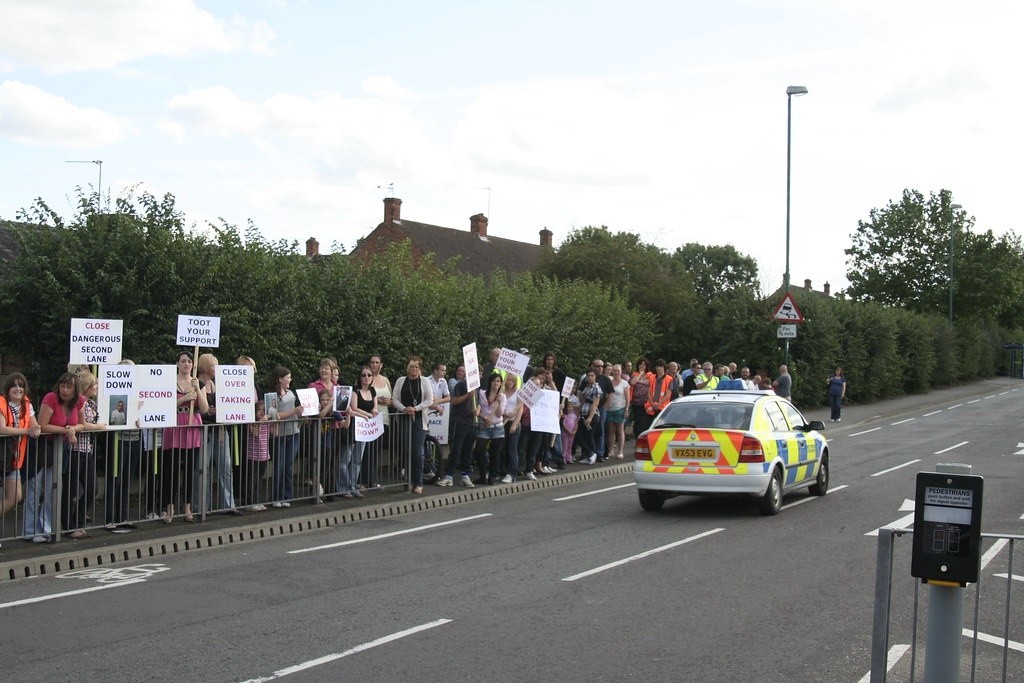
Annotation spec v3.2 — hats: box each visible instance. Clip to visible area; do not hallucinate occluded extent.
[518,347,530,356]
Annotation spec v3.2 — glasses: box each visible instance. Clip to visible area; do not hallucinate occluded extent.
[361,373,372,377]
[704,367,710,370]
[595,365,604,368]
[695,367,701,369]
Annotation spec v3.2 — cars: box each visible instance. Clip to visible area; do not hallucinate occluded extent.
[632,392,832,516]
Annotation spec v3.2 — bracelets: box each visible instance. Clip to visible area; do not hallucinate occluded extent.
[75,427,77,432]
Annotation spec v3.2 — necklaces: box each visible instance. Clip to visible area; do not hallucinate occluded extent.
[409,377,419,405]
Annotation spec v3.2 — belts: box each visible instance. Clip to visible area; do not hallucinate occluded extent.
[480,422,504,428]
[177,406,200,414]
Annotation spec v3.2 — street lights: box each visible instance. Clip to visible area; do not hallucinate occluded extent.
[783,83,810,368]
[949,202,963,324]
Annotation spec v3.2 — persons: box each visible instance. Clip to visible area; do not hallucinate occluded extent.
[667,359,775,402]
[426,348,674,488]
[392,356,434,492]
[773,365,791,402]
[827,367,846,421]
[0,351,280,543]
[306,355,392,501]
[336,388,349,411]
[111,401,126,425]
[268,367,321,507]
[269,399,277,419]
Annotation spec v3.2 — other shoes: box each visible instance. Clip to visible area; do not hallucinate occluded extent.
[31,447,625,544]
[830,419,835,422]
[836,418,841,422]
